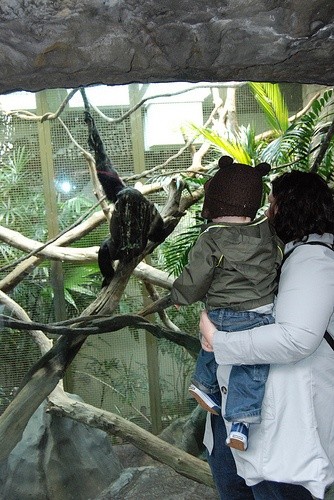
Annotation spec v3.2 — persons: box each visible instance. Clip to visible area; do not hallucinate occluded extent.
[184,167,333,500]
[172,158,282,451]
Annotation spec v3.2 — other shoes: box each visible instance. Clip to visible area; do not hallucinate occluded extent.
[225,420,250,452]
[187,383,222,417]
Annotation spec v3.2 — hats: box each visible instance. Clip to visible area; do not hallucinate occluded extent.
[201,155,271,217]
